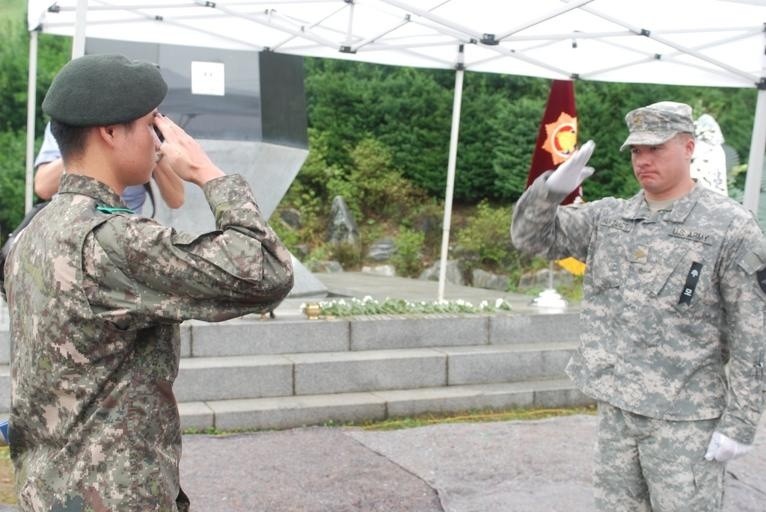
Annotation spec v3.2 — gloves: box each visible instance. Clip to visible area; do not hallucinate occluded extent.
[704,431,752,463]
[546,140,595,195]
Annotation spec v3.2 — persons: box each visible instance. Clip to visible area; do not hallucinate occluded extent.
[0,54,296,511]
[508,102,766,511]
[33,107,186,217]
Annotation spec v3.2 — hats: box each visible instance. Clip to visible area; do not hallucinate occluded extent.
[620,100,694,153]
[42,54,168,126]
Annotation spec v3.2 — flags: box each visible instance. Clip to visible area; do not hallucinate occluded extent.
[526,74,581,207]
